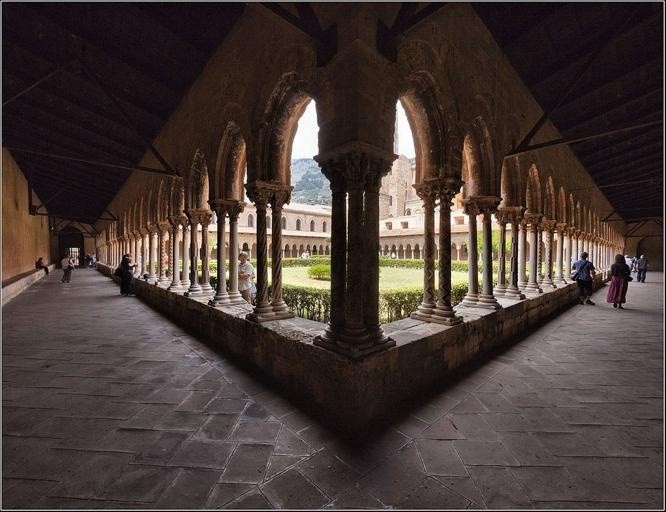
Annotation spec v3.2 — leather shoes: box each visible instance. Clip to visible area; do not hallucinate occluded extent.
[614,304,617,308]
[620,306,624,309]
[587,301,595,305]
[579,301,584,305]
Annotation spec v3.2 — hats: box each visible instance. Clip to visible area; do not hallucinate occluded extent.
[640,254,646,259]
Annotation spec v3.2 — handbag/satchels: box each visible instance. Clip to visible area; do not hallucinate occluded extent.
[570,272,580,281]
[624,274,633,283]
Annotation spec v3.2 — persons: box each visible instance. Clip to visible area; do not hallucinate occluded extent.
[637,254,650,282]
[237,251,256,304]
[60,254,76,283]
[571,251,596,306]
[36,256,48,275]
[625,255,639,273]
[78,252,97,268]
[300,250,310,259]
[116,253,138,298]
[607,254,633,309]
